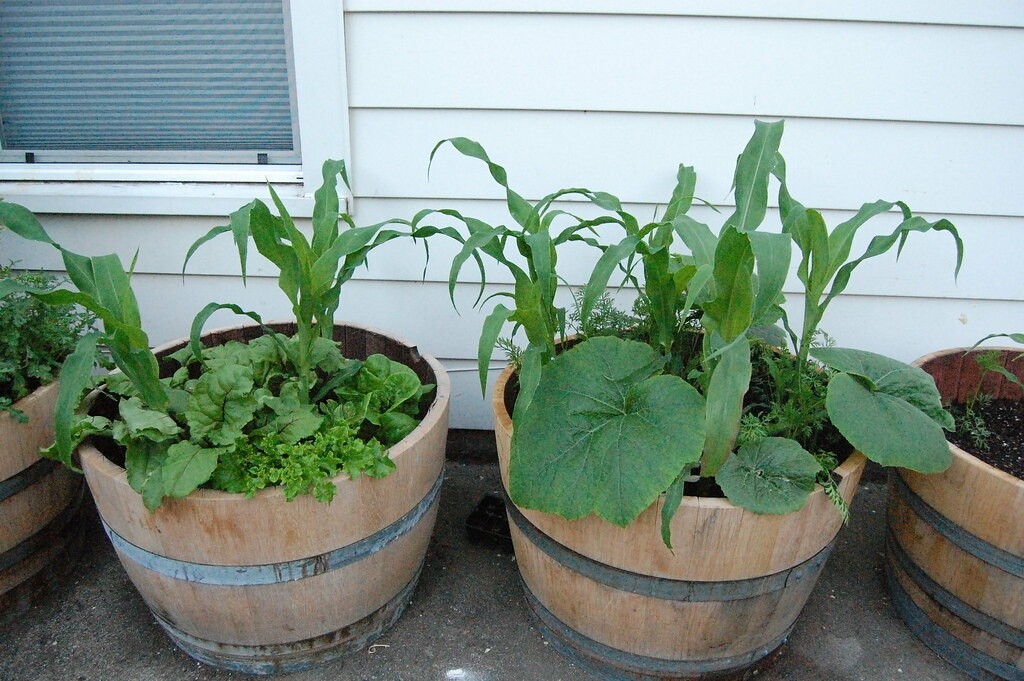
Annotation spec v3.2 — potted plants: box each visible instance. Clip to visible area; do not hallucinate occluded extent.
[0,199,117,612]
[408,118,964,680]
[879,333,1024,680]
[1,158,451,674]
